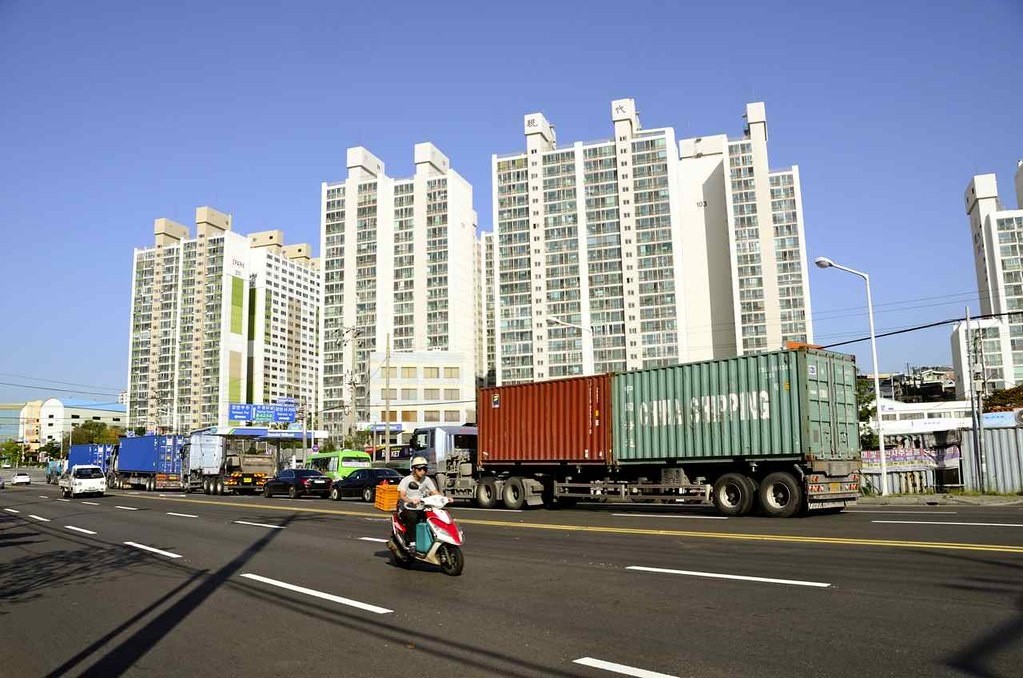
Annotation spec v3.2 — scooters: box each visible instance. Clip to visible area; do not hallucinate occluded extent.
[385,481,464,577]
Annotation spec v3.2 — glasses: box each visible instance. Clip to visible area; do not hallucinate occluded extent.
[416,466,428,471]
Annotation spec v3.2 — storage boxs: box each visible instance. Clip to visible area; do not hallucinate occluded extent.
[375,485,400,512]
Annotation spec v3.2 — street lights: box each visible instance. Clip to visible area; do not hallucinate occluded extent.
[815,257,888,498]
[545,318,594,374]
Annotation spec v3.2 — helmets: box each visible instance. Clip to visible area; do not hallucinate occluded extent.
[411,457,428,468]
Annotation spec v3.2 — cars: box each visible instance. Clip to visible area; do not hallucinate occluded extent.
[263,469,332,499]
[11,472,31,485]
[0,476,5,489]
[329,468,405,502]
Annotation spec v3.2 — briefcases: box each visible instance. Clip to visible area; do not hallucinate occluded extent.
[417,523,433,553]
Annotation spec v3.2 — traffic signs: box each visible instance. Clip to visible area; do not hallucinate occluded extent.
[273,405,295,422]
[252,403,275,423]
[228,403,252,421]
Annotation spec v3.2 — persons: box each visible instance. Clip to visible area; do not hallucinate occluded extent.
[397,457,454,549]
[248,444,257,454]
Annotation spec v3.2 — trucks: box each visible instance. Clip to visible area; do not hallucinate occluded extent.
[43,442,115,485]
[58,464,106,499]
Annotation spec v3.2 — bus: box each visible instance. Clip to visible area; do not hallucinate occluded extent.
[304,449,372,483]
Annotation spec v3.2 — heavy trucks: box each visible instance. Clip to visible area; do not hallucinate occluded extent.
[105,434,185,491]
[409,345,862,518]
[179,434,276,495]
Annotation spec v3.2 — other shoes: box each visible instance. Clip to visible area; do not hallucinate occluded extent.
[409,545,416,552]
